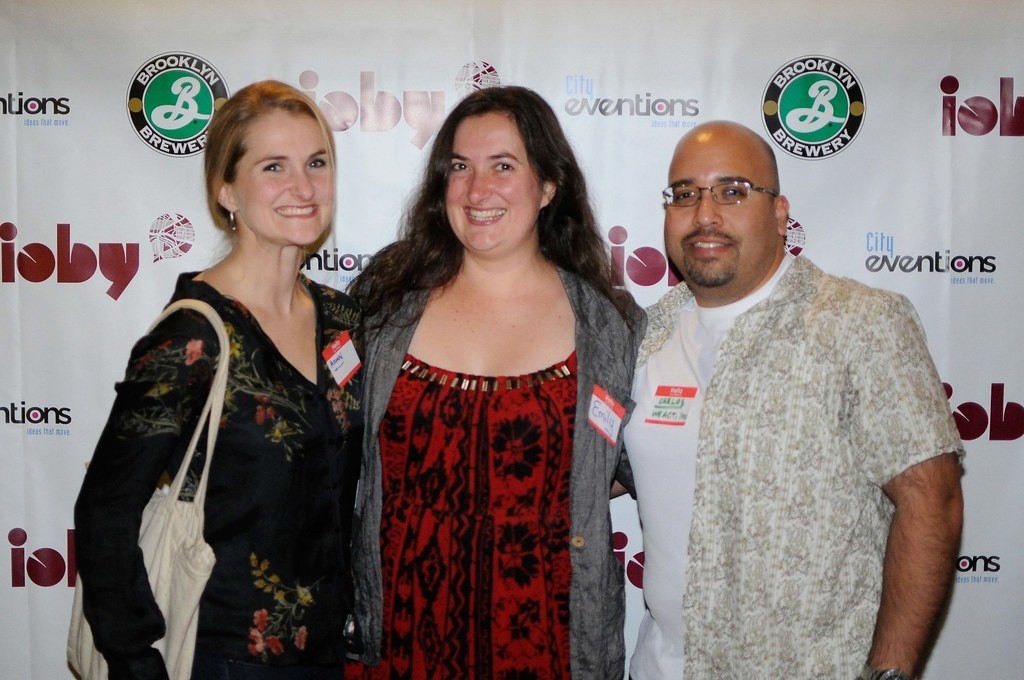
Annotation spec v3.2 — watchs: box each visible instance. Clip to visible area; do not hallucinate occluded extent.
[859,662,910,680]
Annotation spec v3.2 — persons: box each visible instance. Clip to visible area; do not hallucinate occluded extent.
[74,82,364,680]
[610,121,964,680]
[346,85,646,680]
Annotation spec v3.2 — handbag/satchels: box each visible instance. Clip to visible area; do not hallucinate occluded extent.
[66,299,230,680]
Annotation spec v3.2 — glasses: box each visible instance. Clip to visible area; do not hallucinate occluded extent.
[662,181,779,207]
